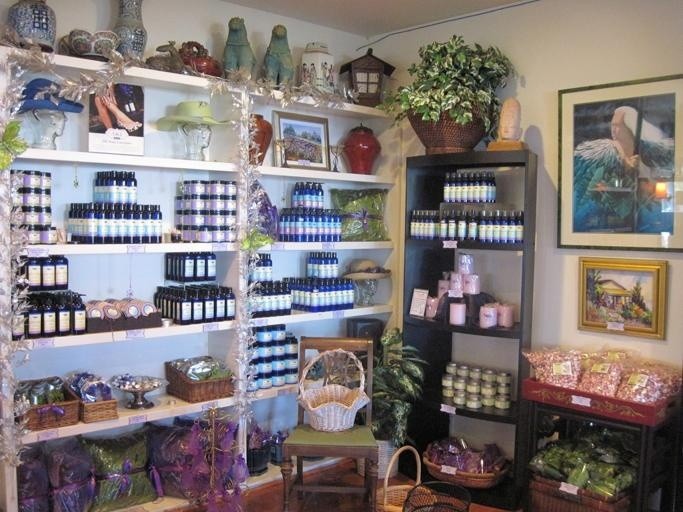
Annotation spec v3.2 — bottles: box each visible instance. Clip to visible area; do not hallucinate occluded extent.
[27,295,41,338]
[409,171,524,244]
[266,430,288,465]
[442,361,512,410]
[41,259,55,289]
[56,293,70,334]
[171,178,237,242]
[42,294,56,337]
[12,296,25,341]
[248,324,298,388]
[249,251,353,317]
[26,256,41,291]
[73,293,87,332]
[279,181,342,242]
[53,255,68,290]
[154,253,235,324]
[7,169,56,244]
[13,256,26,291]
[67,170,162,243]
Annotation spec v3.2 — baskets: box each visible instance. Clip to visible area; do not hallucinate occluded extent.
[531,474,637,512]
[165,356,235,404]
[402,481,473,512]
[17,374,82,429]
[297,348,370,432]
[406,102,492,153]
[376,443,434,512]
[424,448,513,489]
[80,401,120,423]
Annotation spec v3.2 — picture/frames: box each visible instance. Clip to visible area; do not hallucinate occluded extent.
[556,72,683,253]
[577,254,669,342]
[270,109,333,172]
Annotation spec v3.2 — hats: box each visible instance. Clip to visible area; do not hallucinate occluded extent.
[342,258,391,281]
[156,99,232,132]
[19,77,82,114]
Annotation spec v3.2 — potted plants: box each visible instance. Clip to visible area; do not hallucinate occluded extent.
[375,35,517,156]
[356,328,431,480]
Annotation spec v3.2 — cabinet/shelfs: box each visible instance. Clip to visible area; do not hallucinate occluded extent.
[400,148,538,508]
[0,46,407,508]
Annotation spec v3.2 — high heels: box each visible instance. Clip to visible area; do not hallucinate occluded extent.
[115,119,142,132]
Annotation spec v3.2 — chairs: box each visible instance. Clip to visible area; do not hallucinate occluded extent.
[279,336,381,507]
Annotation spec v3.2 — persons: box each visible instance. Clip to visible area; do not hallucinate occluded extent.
[94,85,142,131]
